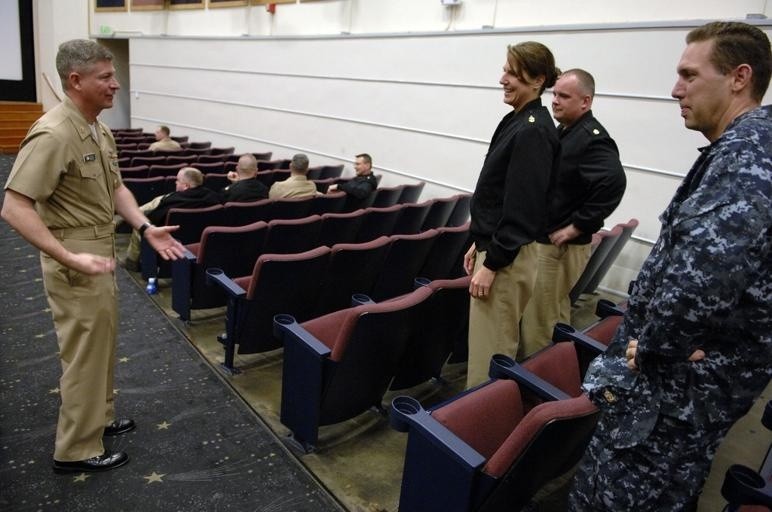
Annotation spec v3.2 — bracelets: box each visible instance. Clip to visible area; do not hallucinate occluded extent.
[138,222,154,235]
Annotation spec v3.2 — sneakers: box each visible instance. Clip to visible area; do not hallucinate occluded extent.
[120,256,138,272]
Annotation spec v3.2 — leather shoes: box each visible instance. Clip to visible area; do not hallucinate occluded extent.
[53,448,129,475]
[102,418,137,436]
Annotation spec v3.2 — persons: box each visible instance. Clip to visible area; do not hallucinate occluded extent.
[1,36,188,474]
[325,153,377,201]
[124,167,217,269]
[519,67,628,352]
[267,153,317,200]
[217,153,267,204]
[148,123,181,151]
[564,22,770,510]
[465,39,559,397]
